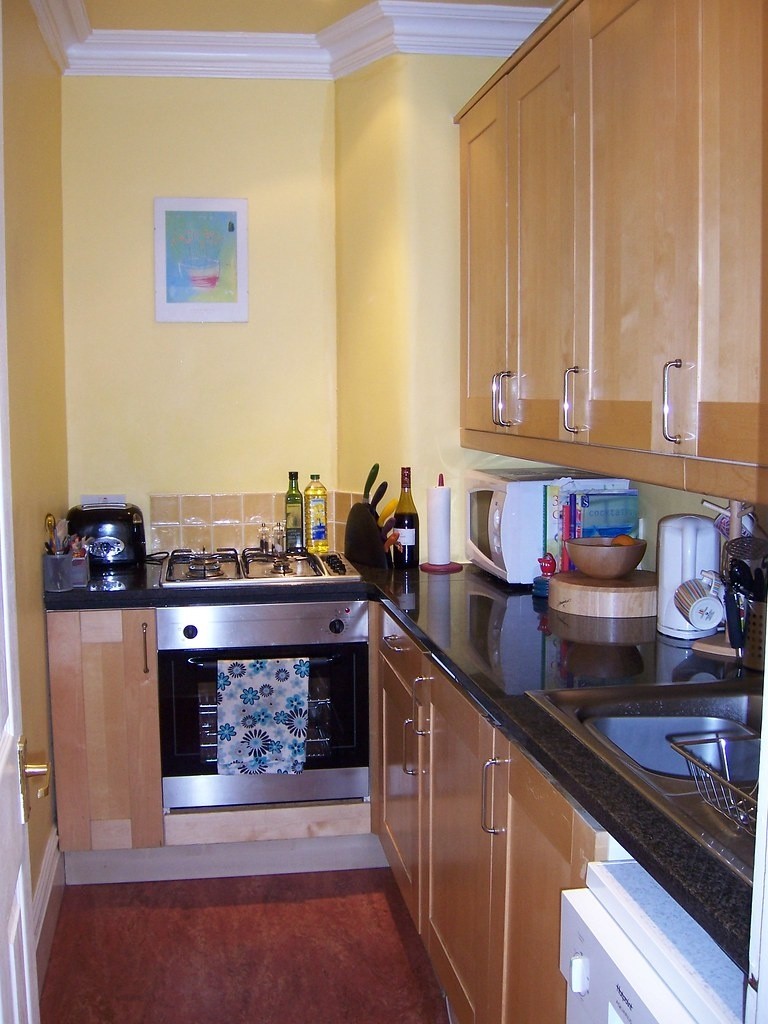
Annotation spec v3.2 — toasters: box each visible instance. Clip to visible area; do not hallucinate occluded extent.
[68,503,147,569]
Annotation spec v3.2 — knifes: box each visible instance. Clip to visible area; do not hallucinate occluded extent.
[362,463,399,552]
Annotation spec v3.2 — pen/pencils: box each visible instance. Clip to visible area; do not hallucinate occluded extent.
[44,536,73,590]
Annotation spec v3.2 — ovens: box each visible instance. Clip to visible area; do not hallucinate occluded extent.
[156,599,370,813]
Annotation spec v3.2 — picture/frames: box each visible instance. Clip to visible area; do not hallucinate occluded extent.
[154,197,248,324]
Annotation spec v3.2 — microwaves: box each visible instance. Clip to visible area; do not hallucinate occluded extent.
[464,567,567,696]
[463,468,630,584]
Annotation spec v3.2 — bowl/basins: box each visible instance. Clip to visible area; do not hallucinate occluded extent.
[563,643,644,677]
[565,538,647,579]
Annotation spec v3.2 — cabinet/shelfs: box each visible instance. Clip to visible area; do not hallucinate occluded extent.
[454,0,768,506]
[372,607,633,1024]
[45,607,165,852]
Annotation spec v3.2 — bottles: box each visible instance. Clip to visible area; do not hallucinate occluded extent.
[394,569,420,623]
[259,523,269,551]
[272,522,284,551]
[393,467,419,568]
[285,471,304,550]
[304,475,329,553]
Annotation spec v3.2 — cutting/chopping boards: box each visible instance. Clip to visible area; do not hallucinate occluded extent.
[547,572,657,618]
[548,609,657,645]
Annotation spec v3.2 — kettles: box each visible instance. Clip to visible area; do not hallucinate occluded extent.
[655,633,724,684]
[656,514,720,638]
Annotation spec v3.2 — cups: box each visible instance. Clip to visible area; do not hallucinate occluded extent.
[673,570,724,630]
[713,505,768,541]
[44,553,73,593]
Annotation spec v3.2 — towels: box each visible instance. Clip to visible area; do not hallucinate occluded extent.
[216,657,310,776]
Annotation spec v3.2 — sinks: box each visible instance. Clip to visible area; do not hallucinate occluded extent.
[522,677,765,798]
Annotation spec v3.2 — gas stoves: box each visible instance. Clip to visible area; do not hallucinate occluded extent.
[159,544,365,605]
